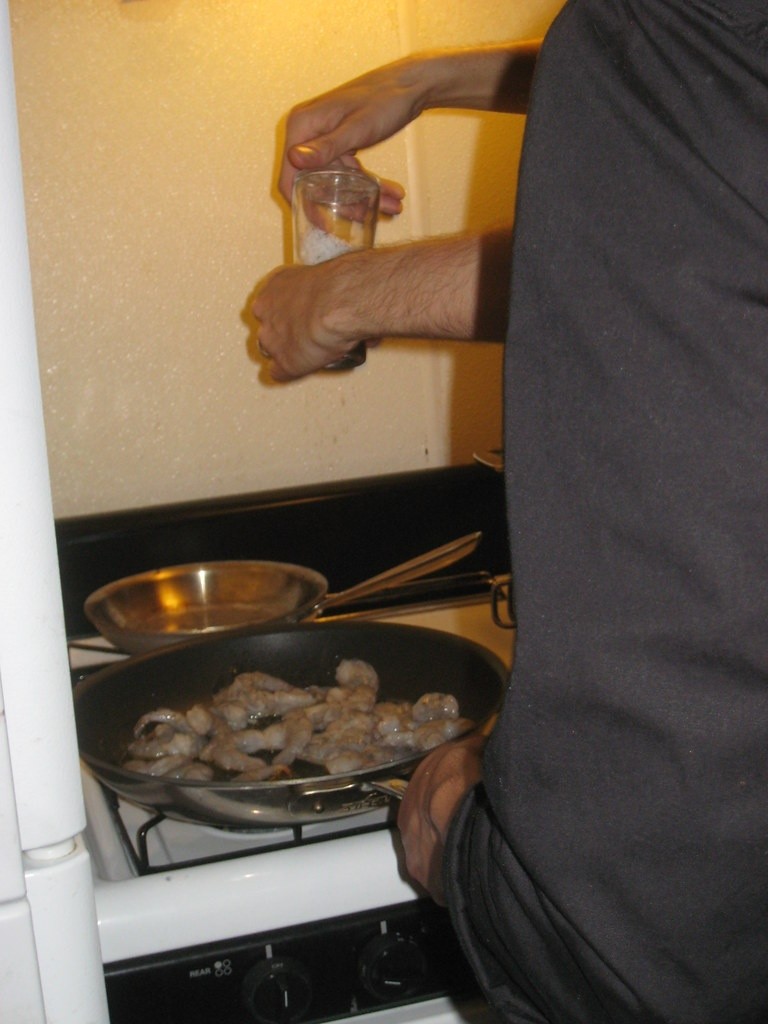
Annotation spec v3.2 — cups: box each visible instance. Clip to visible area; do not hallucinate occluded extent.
[292,167,379,370]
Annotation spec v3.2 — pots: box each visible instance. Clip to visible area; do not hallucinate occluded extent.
[84,531,482,656]
[73,620,510,827]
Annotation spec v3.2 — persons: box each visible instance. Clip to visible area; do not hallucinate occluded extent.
[397,0,768,1024]
[253,38,544,383]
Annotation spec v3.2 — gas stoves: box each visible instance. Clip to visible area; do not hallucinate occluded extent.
[53,463,507,1024]
